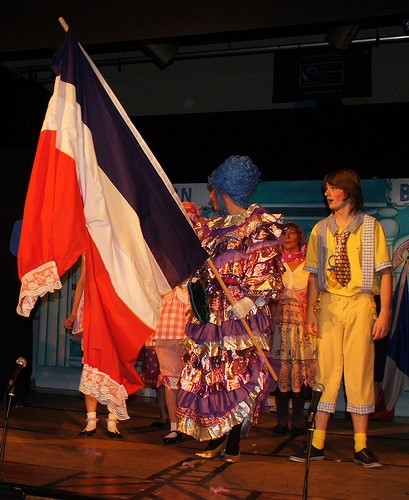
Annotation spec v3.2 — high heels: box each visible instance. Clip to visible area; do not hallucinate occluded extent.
[224,443,240,463]
[196,438,227,459]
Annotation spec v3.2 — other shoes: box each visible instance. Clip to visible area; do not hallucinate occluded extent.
[162,431,182,444]
[150,421,169,429]
[289,443,325,462]
[104,428,123,440]
[291,425,305,435]
[79,428,96,437]
[353,448,383,468]
[274,424,288,435]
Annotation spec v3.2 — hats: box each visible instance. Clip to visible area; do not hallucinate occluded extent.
[206,154,262,216]
[282,222,305,244]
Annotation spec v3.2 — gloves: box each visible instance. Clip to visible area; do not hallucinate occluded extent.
[227,296,254,320]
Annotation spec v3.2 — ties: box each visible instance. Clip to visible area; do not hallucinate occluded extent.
[333,231,351,287]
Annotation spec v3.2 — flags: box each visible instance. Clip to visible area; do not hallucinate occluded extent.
[18,30,211,422]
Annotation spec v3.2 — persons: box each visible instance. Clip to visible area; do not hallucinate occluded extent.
[65,154,393,468]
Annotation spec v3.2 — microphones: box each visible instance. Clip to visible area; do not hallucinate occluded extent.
[8,357,26,386]
[308,383,325,423]
